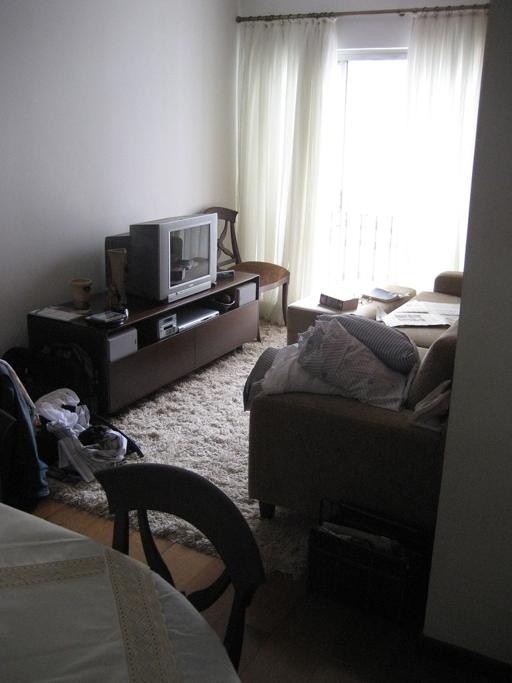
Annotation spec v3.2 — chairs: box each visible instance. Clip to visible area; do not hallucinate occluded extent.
[106,464,264,673]
[204,207,291,341]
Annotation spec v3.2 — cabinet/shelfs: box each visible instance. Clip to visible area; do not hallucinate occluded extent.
[29,270,258,414]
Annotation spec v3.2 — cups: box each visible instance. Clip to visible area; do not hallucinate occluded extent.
[70,277,93,312]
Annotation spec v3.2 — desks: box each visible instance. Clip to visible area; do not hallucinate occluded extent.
[1,500,243,681]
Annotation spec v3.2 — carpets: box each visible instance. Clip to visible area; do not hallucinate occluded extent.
[42,317,308,581]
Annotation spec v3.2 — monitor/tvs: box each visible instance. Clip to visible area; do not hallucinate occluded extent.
[104,212,219,304]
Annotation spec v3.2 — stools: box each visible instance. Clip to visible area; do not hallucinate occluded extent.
[287,286,415,346]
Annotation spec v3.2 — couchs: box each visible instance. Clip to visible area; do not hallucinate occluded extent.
[250,271,465,552]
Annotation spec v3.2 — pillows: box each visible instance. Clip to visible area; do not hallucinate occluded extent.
[318,313,421,376]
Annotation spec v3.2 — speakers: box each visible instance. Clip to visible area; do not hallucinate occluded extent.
[233,281,257,307]
[107,327,138,363]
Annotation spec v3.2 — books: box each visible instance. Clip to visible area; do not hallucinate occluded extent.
[318,289,361,312]
[364,285,410,302]
[170,266,186,280]
[174,258,196,269]
